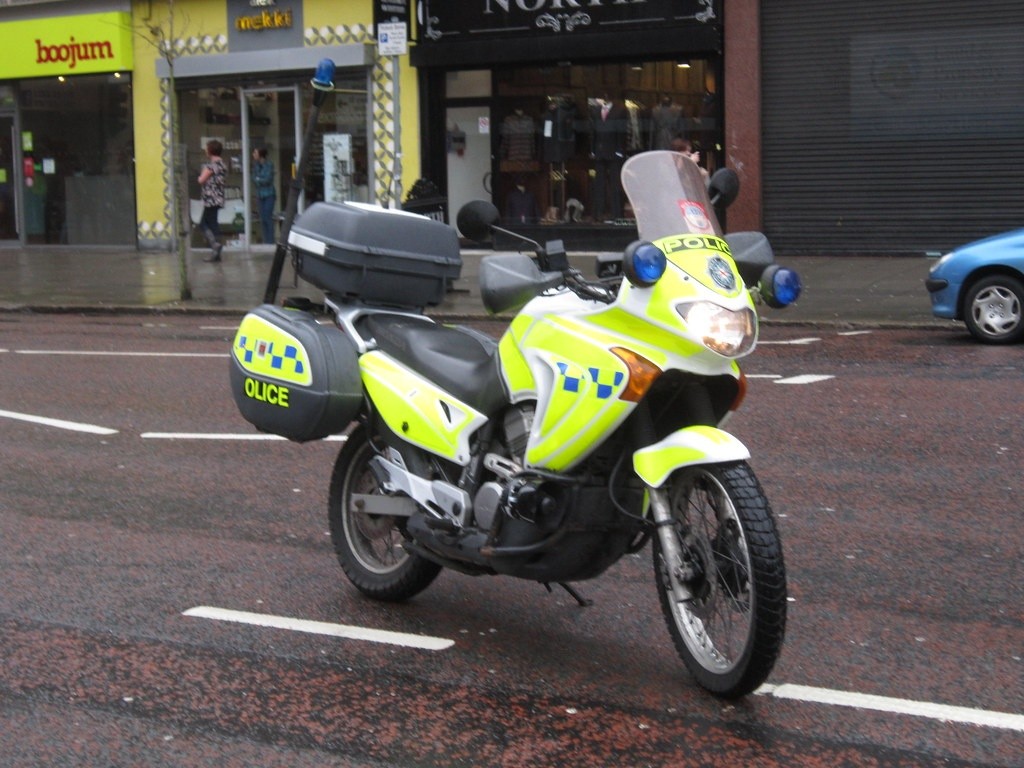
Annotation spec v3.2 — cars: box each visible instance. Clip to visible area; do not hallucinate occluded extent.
[925,226,1024,344]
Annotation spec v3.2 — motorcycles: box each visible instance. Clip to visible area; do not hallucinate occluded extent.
[229,149,801,699]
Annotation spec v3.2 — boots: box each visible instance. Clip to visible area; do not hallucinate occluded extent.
[203,229,222,261]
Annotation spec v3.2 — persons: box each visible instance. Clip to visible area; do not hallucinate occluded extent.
[251,147,275,244]
[501,105,538,223]
[197,140,228,262]
[651,97,684,151]
[588,89,628,222]
